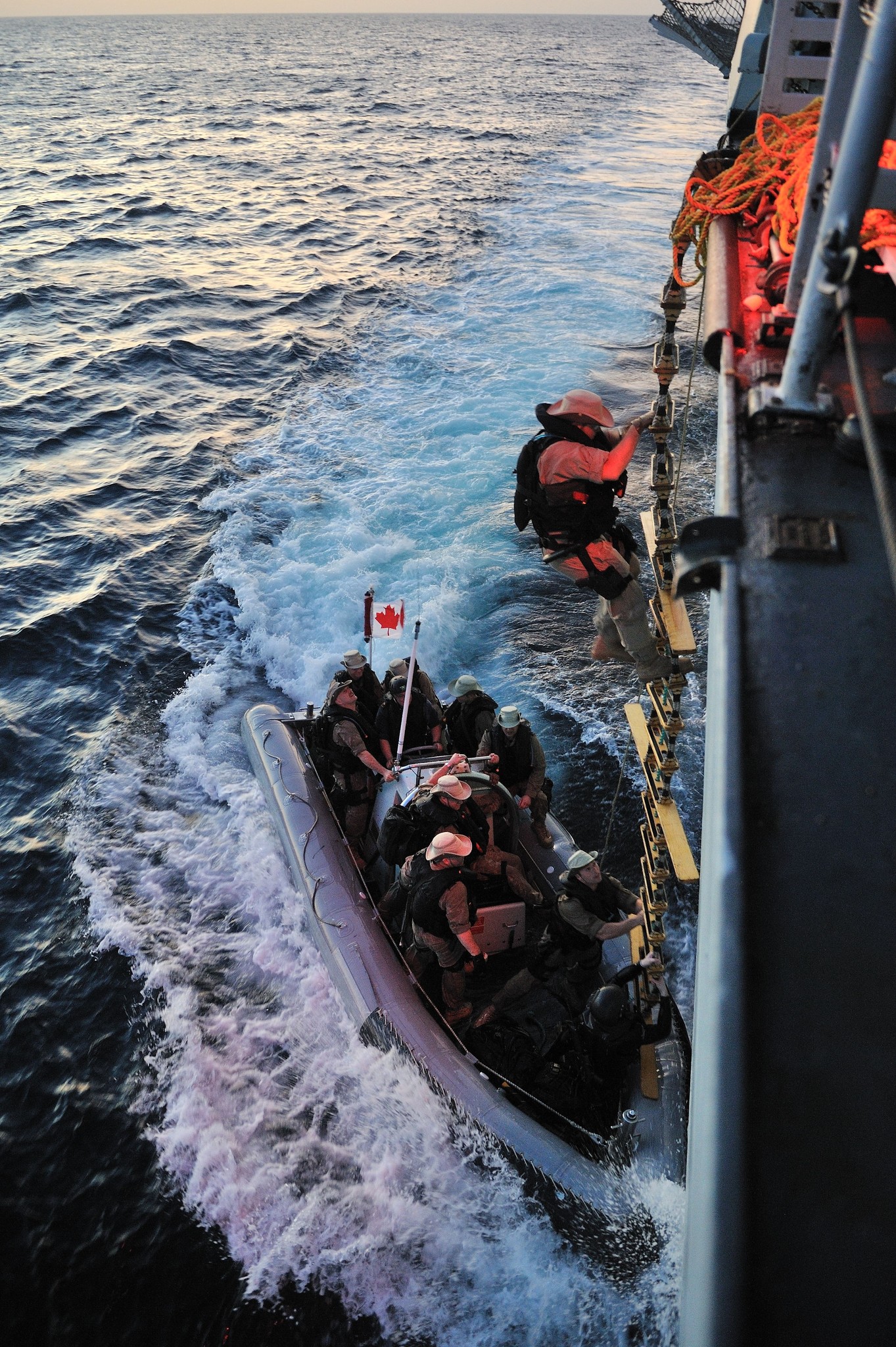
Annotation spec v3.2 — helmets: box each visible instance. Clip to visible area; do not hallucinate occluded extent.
[590,984,628,1027]
[389,675,408,694]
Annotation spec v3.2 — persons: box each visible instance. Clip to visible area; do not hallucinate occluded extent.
[441,673,500,763]
[373,752,477,927]
[373,675,443,770]
[378,657,442,725]
[477,705,556,849]
[407,829,490,1024]
[447,789,545,911]
[330,650,385,729]
[327,680,397,871]
[528,848,649,1011]
[556,949,675,1126]
[532,389,694,683]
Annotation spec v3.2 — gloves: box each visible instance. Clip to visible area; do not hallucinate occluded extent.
[630,411,656,434]
[471,951,486,976]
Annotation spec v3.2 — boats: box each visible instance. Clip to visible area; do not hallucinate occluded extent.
[238,585,690,1237]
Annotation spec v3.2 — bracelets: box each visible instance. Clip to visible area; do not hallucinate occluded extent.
[445,762,451,769]
[432,741,441,744]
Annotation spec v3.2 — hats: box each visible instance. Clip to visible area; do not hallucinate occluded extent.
[327,680,352,706]
[426,832,472,860]
[430,775,472,800]
[546,389,614,428]
[385,658,408,678]
[340,650,367,669]
[448,675,484,698]
[567,850,598,877]
[497,706,521,728]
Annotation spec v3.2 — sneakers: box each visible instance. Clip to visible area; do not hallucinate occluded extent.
[473,1004,499,1029]
[637,656,694,684]
[346,845,367,870]
[592,636,636,662]
[530,819,553,848]
[444,1001,473,1025]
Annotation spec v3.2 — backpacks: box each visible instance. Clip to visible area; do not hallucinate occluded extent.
[376,803,439,867]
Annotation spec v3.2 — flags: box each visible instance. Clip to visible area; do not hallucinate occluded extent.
[365,598,406,642]
